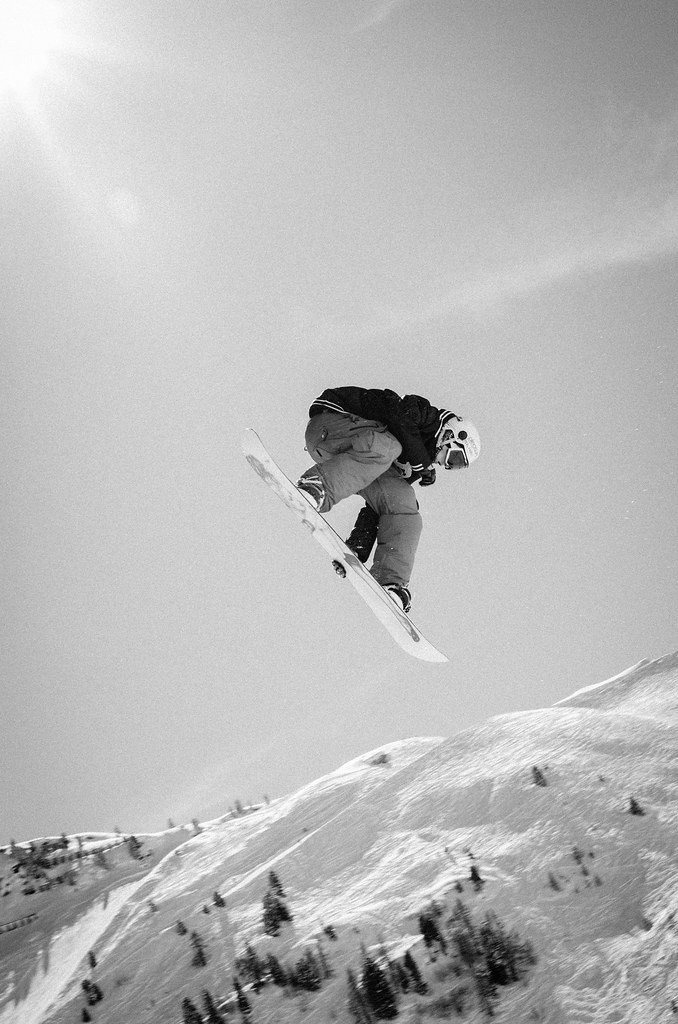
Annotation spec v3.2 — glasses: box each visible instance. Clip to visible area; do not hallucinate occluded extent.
[445,447,468,471]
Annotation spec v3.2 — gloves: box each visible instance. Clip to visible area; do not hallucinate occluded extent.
[332,560,346,578]
[419,464,436,487]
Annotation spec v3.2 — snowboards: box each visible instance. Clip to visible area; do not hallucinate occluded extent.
[235,427,454,667]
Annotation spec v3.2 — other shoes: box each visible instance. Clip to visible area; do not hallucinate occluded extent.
[383,583,413,612]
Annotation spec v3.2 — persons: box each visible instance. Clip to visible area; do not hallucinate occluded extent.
[295,385,481,613]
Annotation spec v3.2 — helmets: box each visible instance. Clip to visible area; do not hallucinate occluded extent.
[436,416,481,468]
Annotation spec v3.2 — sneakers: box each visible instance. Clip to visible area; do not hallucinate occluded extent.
[297,481,325,509]
